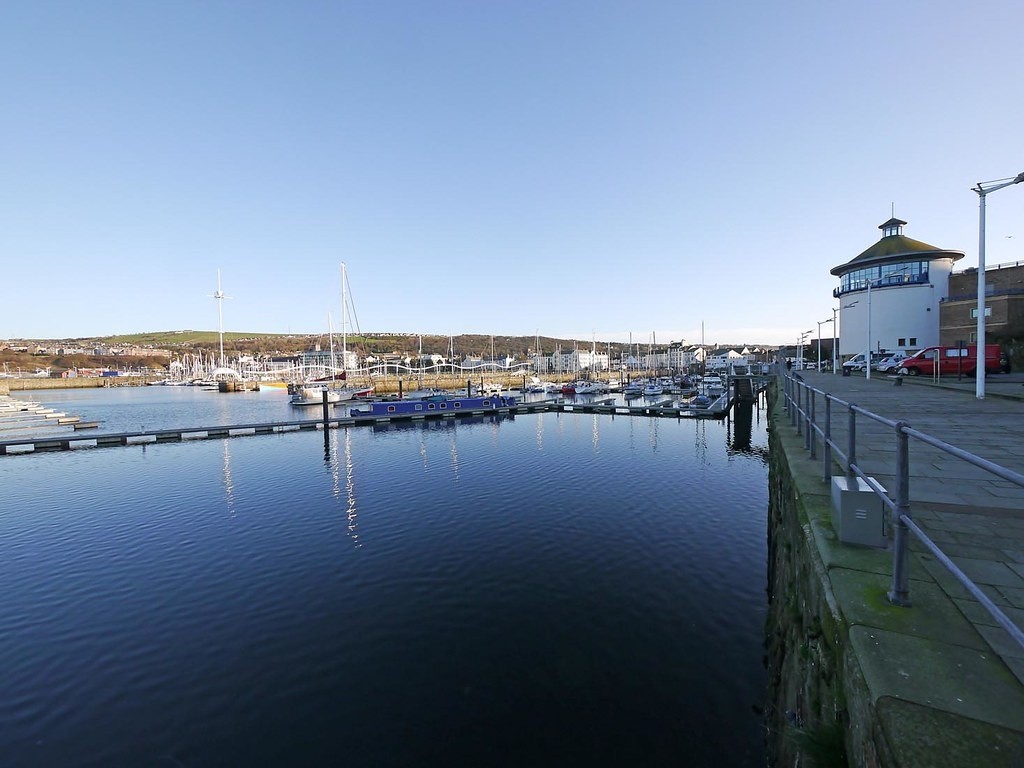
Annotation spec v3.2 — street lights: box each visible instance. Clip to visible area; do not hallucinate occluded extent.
[817,317,837,372]
[796,335,809,370]
[801,329,815,370]
[972,172,1024,400]
[865,266,909,380]
[832,300,859,374]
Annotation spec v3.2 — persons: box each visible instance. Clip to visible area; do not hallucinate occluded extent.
[787,358,792,371]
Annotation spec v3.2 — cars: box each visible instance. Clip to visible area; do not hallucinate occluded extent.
[807,363,816,370]
[855,357,885,373]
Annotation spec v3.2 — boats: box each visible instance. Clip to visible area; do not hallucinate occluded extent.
[146,260,771,438]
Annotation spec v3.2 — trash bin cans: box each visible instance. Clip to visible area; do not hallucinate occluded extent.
[842,366,851,376]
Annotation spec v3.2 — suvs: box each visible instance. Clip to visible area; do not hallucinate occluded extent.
[877,356,910,374]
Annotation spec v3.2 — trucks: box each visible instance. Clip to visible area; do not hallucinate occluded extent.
[843,351,906,373]
[894,344,1002,378]
